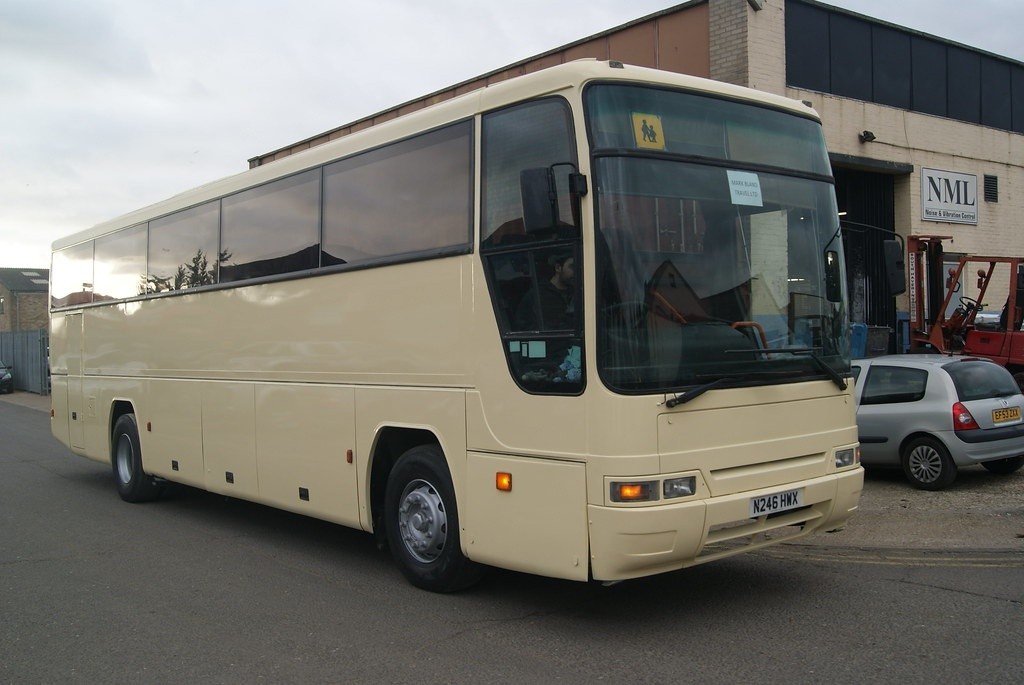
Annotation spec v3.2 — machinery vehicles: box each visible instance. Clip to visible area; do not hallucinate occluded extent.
[908,235,1024,393]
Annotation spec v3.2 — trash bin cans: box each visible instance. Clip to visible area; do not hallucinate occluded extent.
[851,322,868,360]
[791,318,813,352]
[865,323,891,358]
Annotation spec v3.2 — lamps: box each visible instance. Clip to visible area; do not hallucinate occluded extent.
[860,131,876,143]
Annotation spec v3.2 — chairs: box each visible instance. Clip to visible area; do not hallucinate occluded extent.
[864,374,888,402]
[889,372,913,401]
[975,295,1023,331]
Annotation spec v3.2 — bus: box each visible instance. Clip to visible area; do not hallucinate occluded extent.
[49,58,863,595]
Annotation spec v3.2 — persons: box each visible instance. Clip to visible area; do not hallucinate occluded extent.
[514,252,579,364]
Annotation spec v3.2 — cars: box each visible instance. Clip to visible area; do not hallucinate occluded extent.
[0,360,13,393]
[851,354,1024,490]
